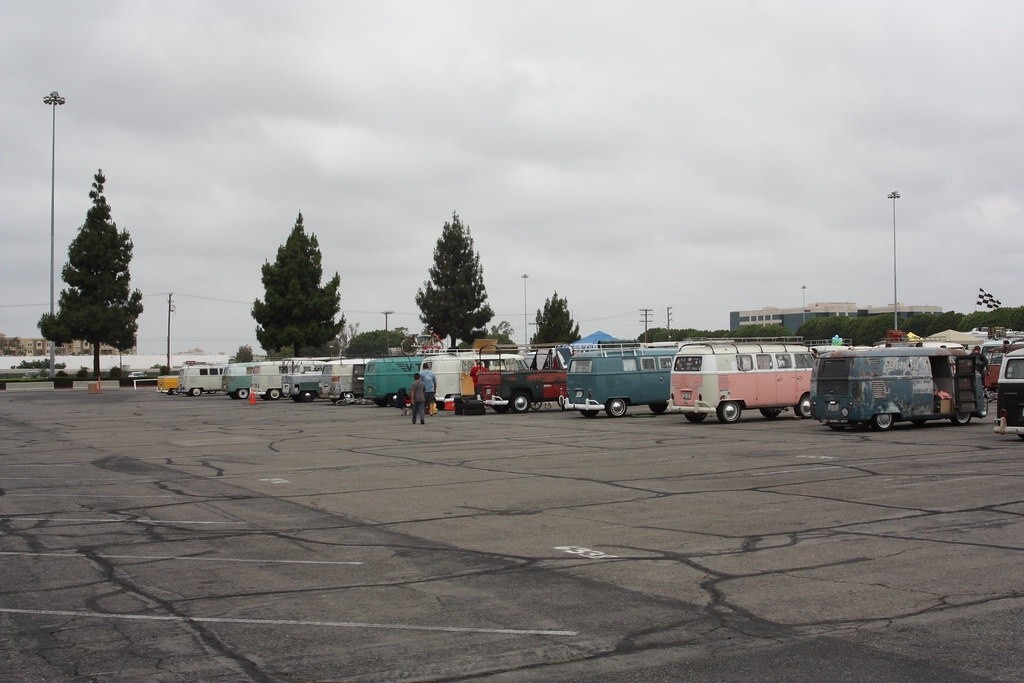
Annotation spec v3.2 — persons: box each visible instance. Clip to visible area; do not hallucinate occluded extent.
[419,363,437,416]
[916,343,922,347]
[410,373,426,424]
[1002,340,1009,351]
[971,346,990,388]
[470,361,486,401]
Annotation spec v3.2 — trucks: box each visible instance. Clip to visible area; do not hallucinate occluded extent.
[158,375,182,395]
[477,343,580,413]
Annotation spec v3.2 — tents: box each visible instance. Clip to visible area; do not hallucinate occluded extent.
[928,330,985,345]
[907,332,923,341]
[575,330,617,348]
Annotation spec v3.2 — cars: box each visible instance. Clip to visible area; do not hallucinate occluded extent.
[128,371,146,376]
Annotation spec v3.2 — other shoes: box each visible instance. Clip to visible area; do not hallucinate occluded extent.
[412,421,415,423]
[420,420,424,424]
[430,413,433,416]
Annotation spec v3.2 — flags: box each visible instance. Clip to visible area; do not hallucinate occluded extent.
[977,288,1001,309]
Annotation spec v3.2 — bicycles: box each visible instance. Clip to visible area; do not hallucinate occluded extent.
[401,327,446,356]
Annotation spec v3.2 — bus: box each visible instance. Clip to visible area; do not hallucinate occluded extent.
[281,359,342,403]
[666,337,818,424]
[319,358,377,405]
[179,366,227,396]
[420,347,529,410]
[223,362,262,400]
[252,362,291,400]
[809,344,987,431]
[564,344,681,417]
[994,347,1024,442]
[981,344,1024,393]
[364,357,435,406]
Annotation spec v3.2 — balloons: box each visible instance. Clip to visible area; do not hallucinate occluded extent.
[832,335,843,346]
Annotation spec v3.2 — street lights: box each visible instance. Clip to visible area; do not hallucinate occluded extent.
[801,285,807,323]
[44,91,65,377]
[888,190,900,331]
[521,274,528,344]
